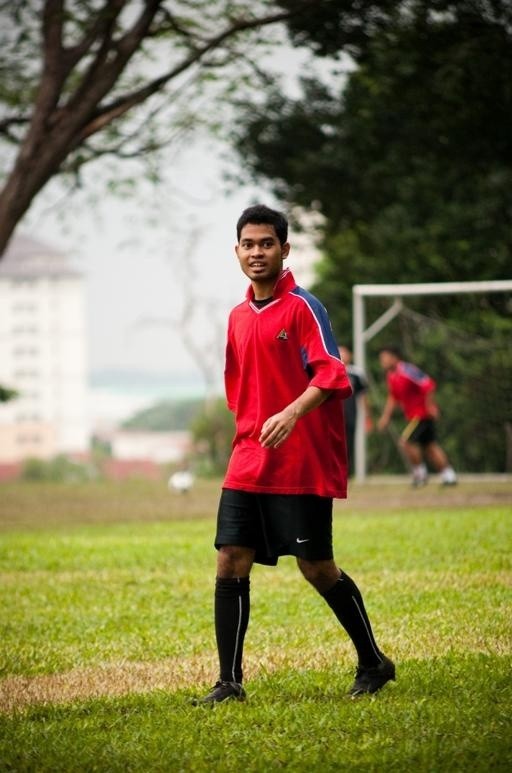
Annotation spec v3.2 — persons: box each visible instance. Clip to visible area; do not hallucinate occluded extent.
[375,348,459,489]
[189,207,396,706]
[338,345,369,476]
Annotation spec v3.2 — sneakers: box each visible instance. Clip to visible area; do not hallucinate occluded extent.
[194,680,246,705]
[409,475,458,488]
[350,661,397,698]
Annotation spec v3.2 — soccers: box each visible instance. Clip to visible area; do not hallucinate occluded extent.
[169,470,197,496]
